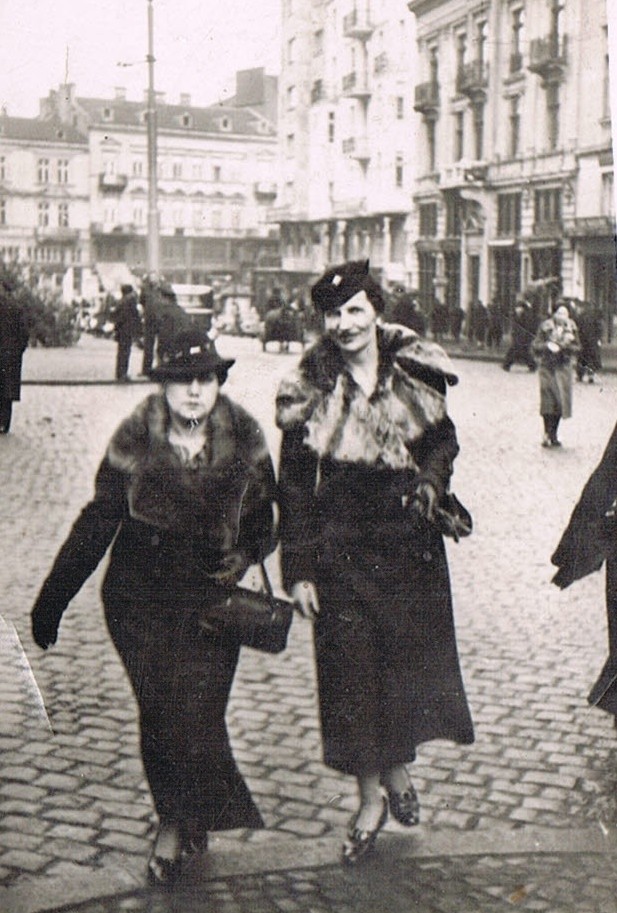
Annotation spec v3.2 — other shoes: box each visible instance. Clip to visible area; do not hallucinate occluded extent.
[542,434,549,446]
[552,434,559,444]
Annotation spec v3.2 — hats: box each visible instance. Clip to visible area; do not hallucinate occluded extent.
[312,259,370,310]
[147,331,235,376]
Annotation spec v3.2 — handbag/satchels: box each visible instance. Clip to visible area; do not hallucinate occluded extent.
[435,493,473,542]
[542,342,563,366]
[224,561,293,654]
[552,514,614,590]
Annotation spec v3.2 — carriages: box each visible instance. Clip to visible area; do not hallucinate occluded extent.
[257,266,321,357]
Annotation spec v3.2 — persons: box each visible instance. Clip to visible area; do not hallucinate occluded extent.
[0,278,30,434]
[550,425,617,728]
[30,334,278,887]
[113,273,177,384]
[265,274,604,451]
[274,260,474,866]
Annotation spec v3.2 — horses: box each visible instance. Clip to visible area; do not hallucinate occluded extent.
[277,297,294,352]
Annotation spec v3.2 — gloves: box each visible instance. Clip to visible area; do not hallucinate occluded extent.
[30,605,60,648]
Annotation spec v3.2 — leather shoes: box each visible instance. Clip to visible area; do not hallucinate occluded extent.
[341,795,388,863]
[147,821,184,889]
[381,764,422,824]
[184,829,208,854]
